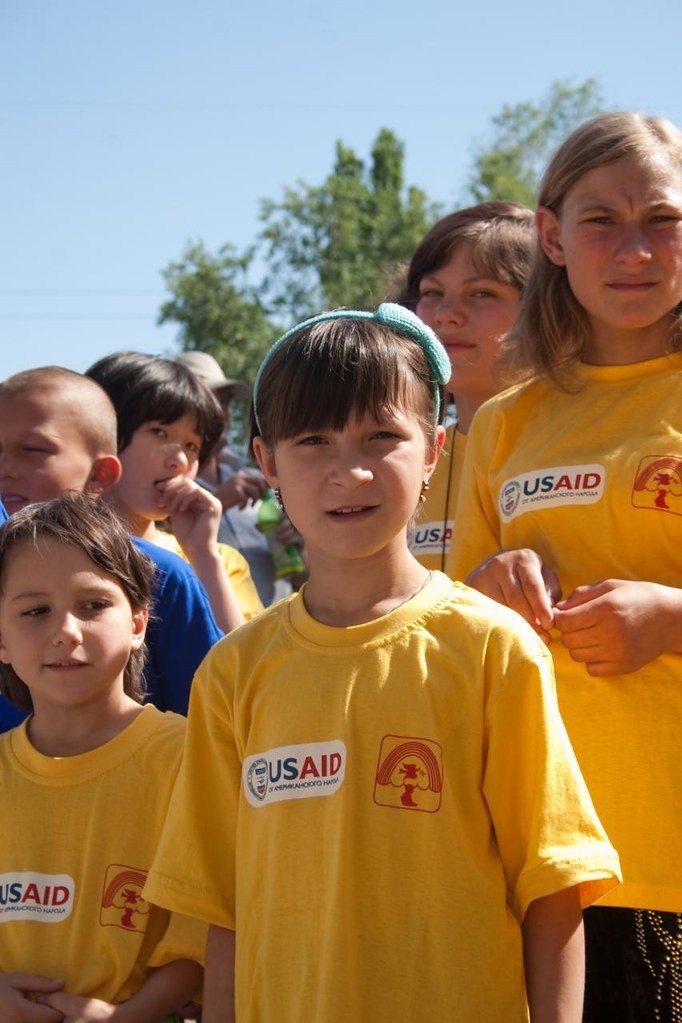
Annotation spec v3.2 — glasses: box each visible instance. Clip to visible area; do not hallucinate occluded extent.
[215,393,235,405]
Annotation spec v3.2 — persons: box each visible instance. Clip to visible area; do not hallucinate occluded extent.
[0,488,211,1023]
[446,111,682,1022]
[0,346,307,731]
[141,302,621,1023]
[397,203,543,575]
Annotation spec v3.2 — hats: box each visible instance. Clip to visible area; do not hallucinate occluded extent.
[173,350,247,399]
